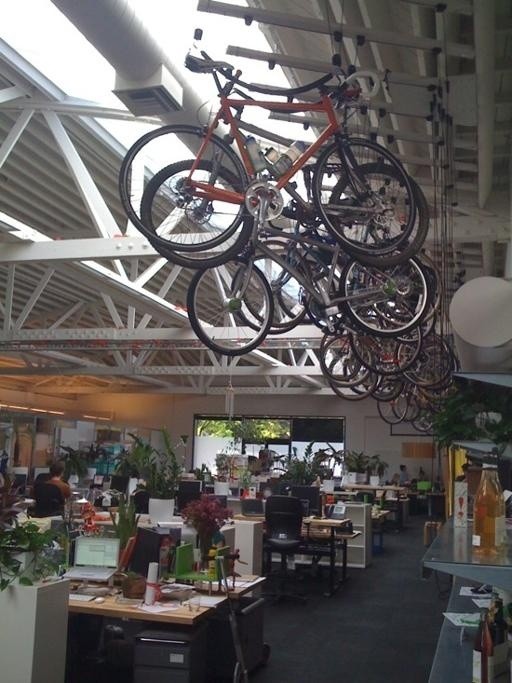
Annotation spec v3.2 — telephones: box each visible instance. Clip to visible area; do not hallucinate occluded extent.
[327,505,346,519]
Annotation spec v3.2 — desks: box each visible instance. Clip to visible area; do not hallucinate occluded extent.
[0,482,444,683]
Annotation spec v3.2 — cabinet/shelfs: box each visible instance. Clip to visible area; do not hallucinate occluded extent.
[420,370,512,683]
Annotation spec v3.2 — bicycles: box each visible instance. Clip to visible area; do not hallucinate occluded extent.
[117,53,464,433]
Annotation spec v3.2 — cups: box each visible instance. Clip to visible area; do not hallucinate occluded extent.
[188,594,200,614]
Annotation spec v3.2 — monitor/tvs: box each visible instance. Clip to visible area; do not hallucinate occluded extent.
[62,537,119,581]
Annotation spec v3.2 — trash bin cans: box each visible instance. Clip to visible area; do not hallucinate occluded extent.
[372,529,381,556]
[214,596,270,682]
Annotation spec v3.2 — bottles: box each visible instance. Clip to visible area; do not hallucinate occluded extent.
[472,591,508,683]
[471,453,507,555]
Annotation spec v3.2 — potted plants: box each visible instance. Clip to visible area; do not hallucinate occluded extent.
[344,449,389,485]
[57,442,107,485]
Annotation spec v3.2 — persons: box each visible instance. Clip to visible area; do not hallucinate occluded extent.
[456,461,474,482]
[399,463,409,486]
[34,461,72,517]
